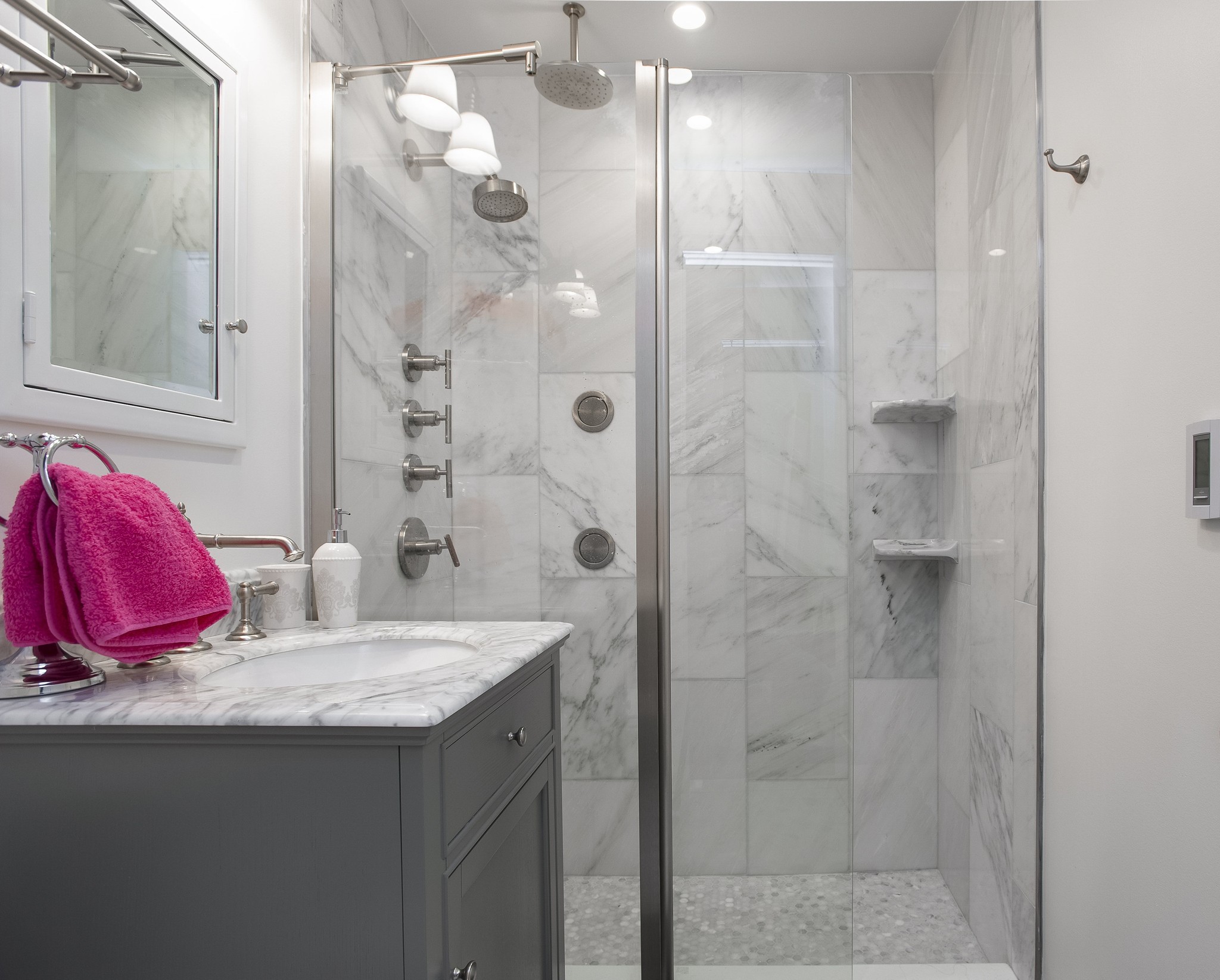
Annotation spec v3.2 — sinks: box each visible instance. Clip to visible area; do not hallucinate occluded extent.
[179,625,492,686]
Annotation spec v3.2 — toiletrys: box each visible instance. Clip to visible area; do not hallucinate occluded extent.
[311,507,363,630]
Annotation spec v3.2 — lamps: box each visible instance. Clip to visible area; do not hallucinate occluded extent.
[402,111,503,180]
[388,60,463,133]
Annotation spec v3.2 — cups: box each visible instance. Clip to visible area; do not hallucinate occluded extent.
[255,560,312,629]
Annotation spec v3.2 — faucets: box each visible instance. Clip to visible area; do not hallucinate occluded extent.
[169,501,305,562]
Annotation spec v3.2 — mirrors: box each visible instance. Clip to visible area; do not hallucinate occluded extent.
[18,0,248,418]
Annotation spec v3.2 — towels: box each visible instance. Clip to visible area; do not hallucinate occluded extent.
[1,462,233,664]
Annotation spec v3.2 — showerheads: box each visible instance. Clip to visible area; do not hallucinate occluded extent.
[533,0,614,111]
[401,137,530,223]
[332,40,544,85]
